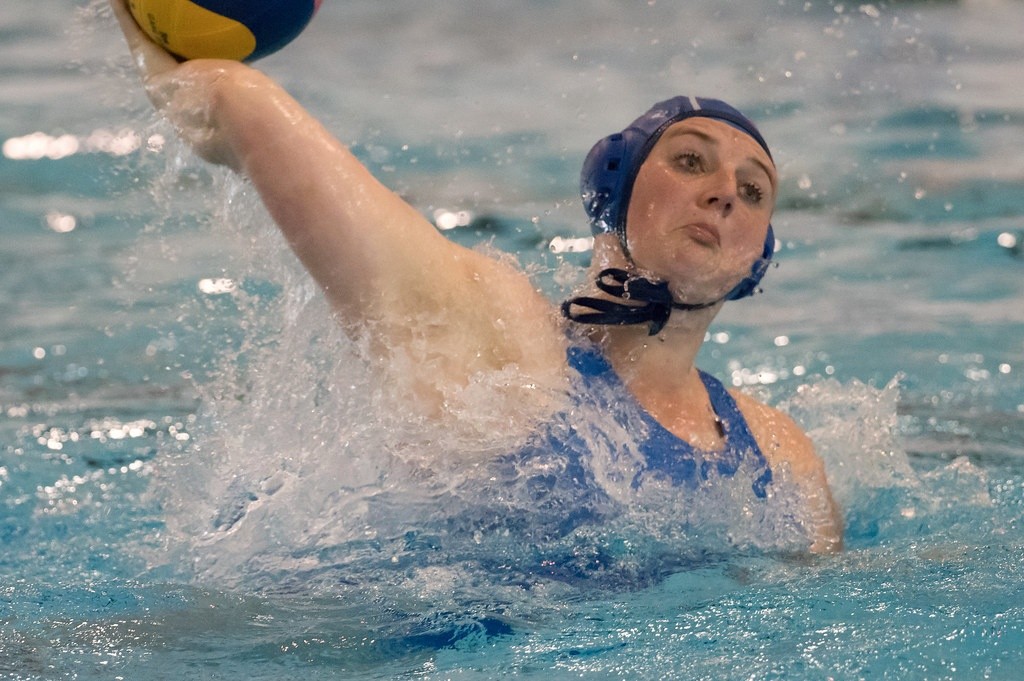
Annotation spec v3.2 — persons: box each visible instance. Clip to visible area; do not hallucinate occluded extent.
[111,1,844,591]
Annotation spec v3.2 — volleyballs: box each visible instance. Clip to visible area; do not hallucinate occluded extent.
[122,0,325,67]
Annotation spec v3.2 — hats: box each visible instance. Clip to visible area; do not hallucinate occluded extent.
[558,93,776,337]
